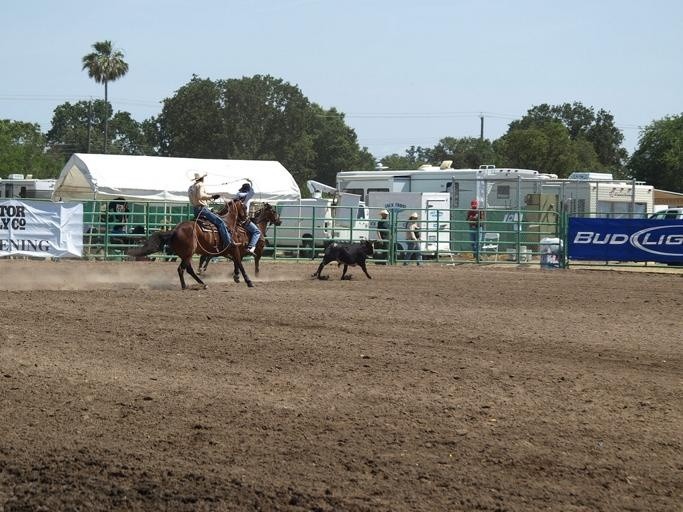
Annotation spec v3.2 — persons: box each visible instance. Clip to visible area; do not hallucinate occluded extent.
[232,176,261,256]
[464,200,482,258]
[109,225,126,250]
[400,211,423,266]
[375,208,389,266]
[185,170,242,249]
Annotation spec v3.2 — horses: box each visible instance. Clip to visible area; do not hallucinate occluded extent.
[126,194,254,298]
[196,201,283,277]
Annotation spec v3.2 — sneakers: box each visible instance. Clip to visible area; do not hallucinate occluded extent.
[246,247,256,256]
[476,259,482,262]
[417,263,423,266]
[223,250,232,254]
[473,254,476,258]
[404,264,409,266]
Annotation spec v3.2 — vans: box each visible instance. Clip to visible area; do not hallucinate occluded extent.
[646,207,682,220]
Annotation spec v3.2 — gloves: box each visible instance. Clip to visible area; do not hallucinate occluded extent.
[212,195,220,199]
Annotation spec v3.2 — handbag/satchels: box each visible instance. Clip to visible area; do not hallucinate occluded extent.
[414,228,421,238]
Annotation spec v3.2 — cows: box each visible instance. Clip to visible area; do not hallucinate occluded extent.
[311,239,378,280]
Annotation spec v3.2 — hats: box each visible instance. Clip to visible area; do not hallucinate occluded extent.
[380,210,389,214]
[471,200,477,207]
[239,183,250,192]
[411,212,418,217]
[191,173,207,181]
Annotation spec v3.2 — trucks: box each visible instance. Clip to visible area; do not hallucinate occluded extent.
[250,196,370,260]
